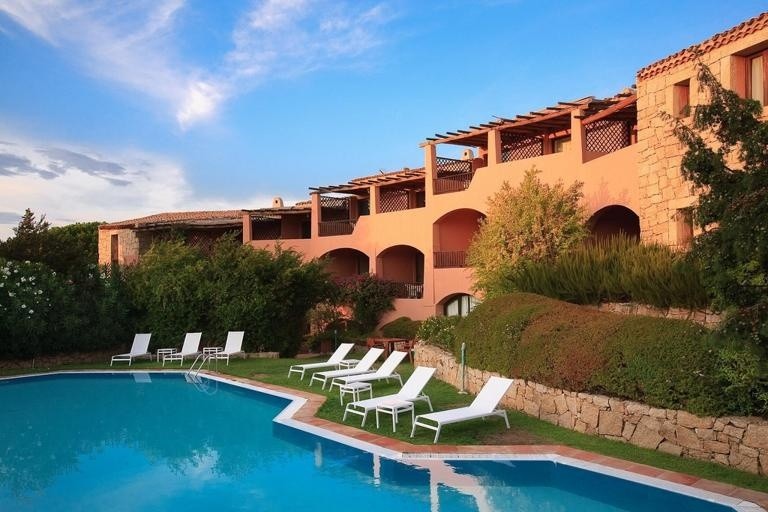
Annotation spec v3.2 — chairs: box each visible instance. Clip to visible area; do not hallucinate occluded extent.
[109,331,247,367]
[287,343,515,445]
